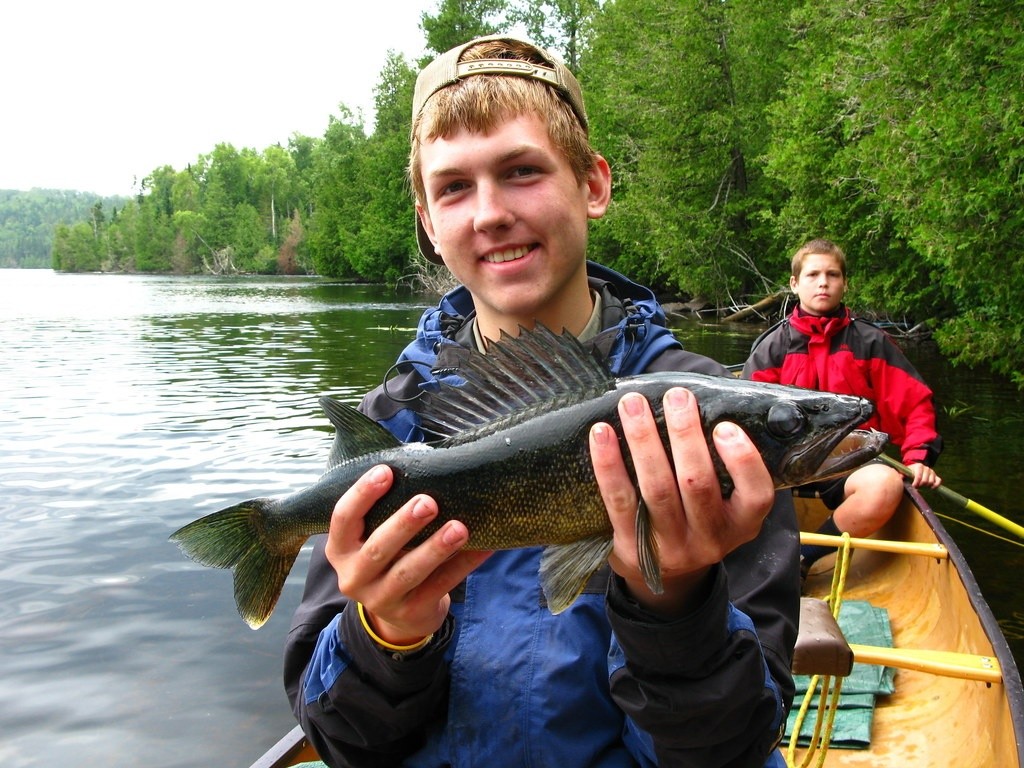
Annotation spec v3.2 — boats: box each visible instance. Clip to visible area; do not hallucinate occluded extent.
[237,431,1024,767]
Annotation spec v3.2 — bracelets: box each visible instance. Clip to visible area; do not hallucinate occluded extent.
[358,601,433,653]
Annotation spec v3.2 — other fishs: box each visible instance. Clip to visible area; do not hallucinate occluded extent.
[167,316,892,632]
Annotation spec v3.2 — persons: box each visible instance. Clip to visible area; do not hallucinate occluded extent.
[284,33,802,768]
[739,238,946,583]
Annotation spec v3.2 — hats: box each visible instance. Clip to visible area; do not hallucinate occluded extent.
[410,36,588,268]
